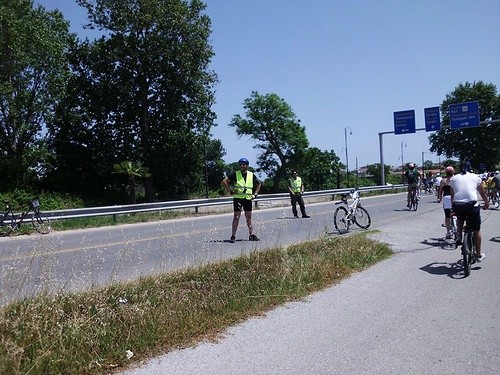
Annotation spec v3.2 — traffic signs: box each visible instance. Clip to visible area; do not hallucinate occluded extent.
[424,107,441,132]
[393,109,416,135]
[449,101,480,130]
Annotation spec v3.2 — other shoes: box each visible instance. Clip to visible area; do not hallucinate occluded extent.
[446,234,451,238]
[249,234,260,241]
[477,253,485,263]
[294,215,299,218]
[456,237,461,243]
[230,235,235,243]
[302,214,310,218]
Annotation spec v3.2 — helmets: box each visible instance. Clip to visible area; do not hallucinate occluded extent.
[239,158,249,165]
[437,173,440,176]
[445,166,455,174]
[494,171,500,173]
[409,164,414,168]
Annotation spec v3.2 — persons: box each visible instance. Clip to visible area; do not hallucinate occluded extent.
[288,170,310,218]
[223,158,262,243]
[405,161,500,262]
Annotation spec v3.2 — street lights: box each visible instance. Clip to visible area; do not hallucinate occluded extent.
[342,126,352,187]
[422,151,433,170]
[398,140,407,186]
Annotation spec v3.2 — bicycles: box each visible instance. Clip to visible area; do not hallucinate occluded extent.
[438,200,484,276]
[410,186,418,211]
[334,183,371,234]
[0,193,51,237]
[425,181,500,208]
